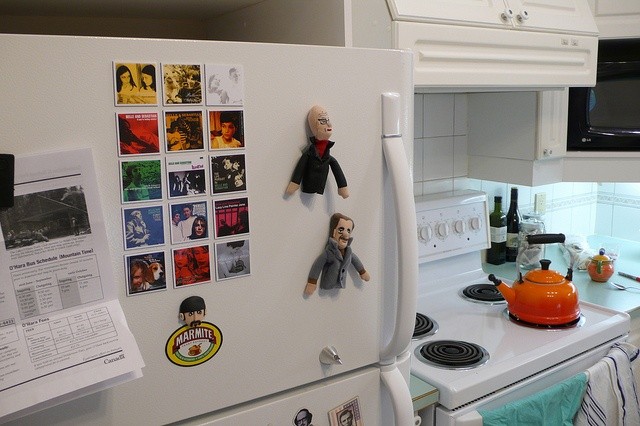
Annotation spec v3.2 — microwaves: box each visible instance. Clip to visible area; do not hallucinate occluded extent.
[566,38,640,151]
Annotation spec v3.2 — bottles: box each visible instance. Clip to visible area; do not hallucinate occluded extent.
[487,195,507,266]
[507,187,523,263]
[518,213,545,270]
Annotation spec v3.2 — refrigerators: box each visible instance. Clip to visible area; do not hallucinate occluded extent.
[0,32,418,426]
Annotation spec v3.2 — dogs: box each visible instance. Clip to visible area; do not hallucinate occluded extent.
[147,263,165,284]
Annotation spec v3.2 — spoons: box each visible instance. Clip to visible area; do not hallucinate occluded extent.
[611,283,640,291]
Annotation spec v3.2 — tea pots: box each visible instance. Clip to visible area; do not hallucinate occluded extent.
[489,234,581,325]
[588,248,616,283]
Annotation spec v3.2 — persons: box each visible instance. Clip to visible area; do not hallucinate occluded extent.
[167,121,183,151]
[118,117,159,154]
[139,64,156,104]
[227,68,242,104]
[294,408,313,426]
[114,62,139,104]
[232,159,245,188]
[304,213,370,296]
[207,73,227,105]
[193,173,203,192]
[173,175,183,192]
[219,210,249,236]
[172,212,184,243]
[191,215,206,239]
[288,105,350,200]
[217,240,247,278]
[124,165,149,201]
[183,173,192,190]
[71,216,81,236]
[339,409,354,426]
[223,158,240,189]
[176,70,203,104]
[179,296,207,327]
[126,211,151,246]
[5,227,49,248]
[130,258,150,294]
[173,248,195,286]
[178,117,190,150]
[211,111,241,149]
[195,245,211,284]
[181,206,197,240]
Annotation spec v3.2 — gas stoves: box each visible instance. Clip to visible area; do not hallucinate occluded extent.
[409,189,631,410]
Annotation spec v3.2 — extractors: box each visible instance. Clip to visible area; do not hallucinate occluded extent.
[350,0,598,94]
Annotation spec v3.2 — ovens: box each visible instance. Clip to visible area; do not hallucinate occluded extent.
[433,333,635,426]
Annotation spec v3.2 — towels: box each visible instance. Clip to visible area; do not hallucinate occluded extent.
[573,342,640,426]
[481,370,587,426]
